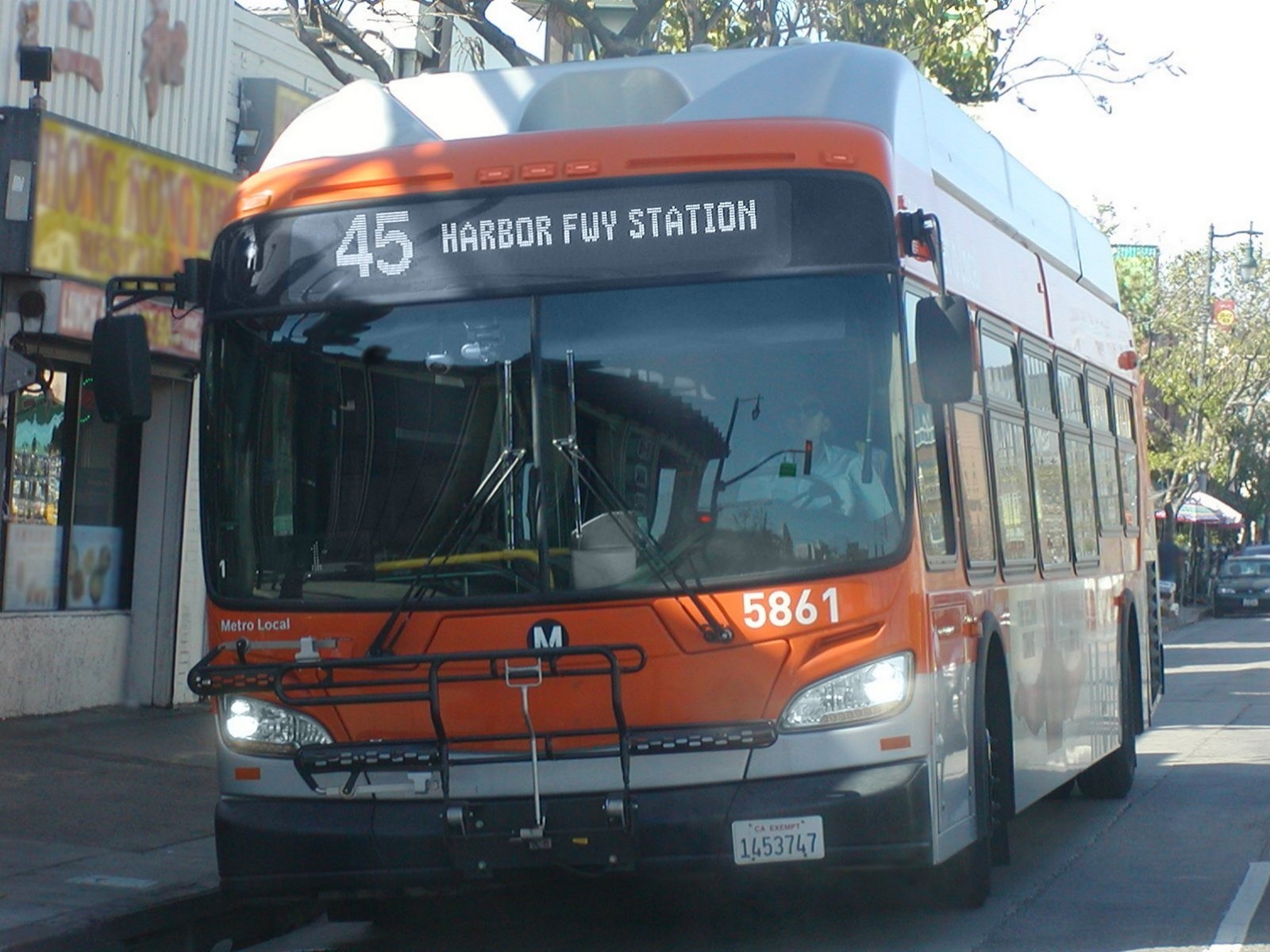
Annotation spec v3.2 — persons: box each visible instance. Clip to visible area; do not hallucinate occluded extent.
[1158,531,1184,601]
[729,381,902,566]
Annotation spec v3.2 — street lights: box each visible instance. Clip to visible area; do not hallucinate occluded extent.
[1190,221,1264,607]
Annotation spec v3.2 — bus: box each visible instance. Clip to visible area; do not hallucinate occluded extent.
[88,38,1167,951]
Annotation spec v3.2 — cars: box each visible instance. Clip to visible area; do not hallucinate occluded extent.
[1209,544,1270,618]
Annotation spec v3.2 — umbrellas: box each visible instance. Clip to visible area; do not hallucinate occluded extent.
[1155,490,1243,604]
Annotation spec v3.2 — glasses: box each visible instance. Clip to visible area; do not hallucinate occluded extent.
[782,401,825,417]
